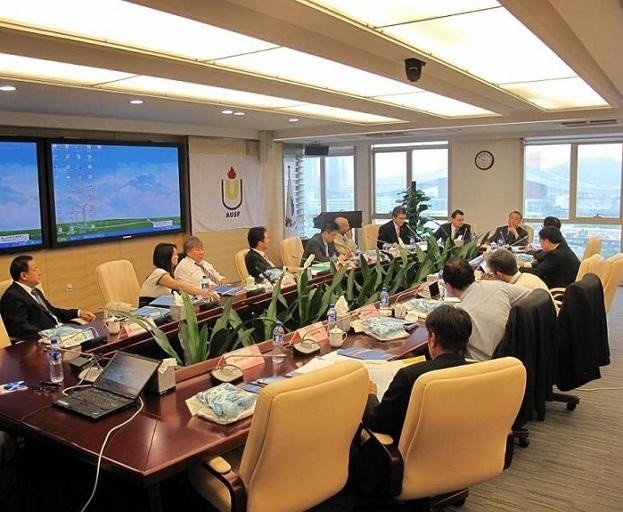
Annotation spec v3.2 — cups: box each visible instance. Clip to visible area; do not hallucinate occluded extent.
[394,304,408,321]
[490,241,497,250]
[475,269,484,281]
[326,327,349,350]
[299,266,313,282]
[243,274,256,289]
[102,315,123,336]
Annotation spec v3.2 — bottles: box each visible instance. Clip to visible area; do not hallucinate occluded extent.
[48,337,67,383]
[354,245,360,259]
[326,303,336,338]
[270,318,288,365]
[409,234,416,251]
[201,273,212,303]
[382,241,388,253]
[437,266,445,290]
[380,285,390,310]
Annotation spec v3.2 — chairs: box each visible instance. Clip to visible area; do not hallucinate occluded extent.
[361,355,525,510]
[543,272,611,411]
[550,253,622,316]
[279,237,320,272]
[520,224,534,245]
[189,359,369,512]
[236,250,251,282]
[361,224,382,250]
[553,254,605,299]
[581,234,602,261]
[0,280,48,347]
[93,260,142,309]
[488,288,558,446]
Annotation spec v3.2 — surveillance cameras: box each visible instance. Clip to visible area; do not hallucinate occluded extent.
[404,58,425,82]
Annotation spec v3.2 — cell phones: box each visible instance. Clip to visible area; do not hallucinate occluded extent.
[69,354,94,367]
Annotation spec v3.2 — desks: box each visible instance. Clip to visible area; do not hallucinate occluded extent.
[0,244,543,512]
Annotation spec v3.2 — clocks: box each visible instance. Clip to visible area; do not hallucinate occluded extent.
[474,150,494,170]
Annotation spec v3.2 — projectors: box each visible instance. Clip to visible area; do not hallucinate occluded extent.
[305,144,329,156]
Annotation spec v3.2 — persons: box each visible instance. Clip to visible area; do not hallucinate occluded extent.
[433,209,471,242]
[139,243,220,309]
[334,216,357,256]
[518,227,578,297]
[525,216,578,257]
[363,305,478,433]
[175,237,228,294]
[444,258,532,361]
[300,222,339,267]
[1,255,96,343]
[484,251,559,315]
[489,211,529,246]
[246,227,299,283]
[377,206,412,248]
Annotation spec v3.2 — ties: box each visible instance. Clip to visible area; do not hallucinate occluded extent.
[455,228,458,234]
[30,289,60,321]
[194,261,221,287]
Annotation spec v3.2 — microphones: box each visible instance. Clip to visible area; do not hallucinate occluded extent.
[406,224,422,240]
[462,228,467,240]
[500,231,506,244]
[211,353,287,383]
[53,346,103,382]
[510,235,529,247]
[391,287,423,313]
[293,311,361,356]
[292,256,331,276]
[367,235,387,243]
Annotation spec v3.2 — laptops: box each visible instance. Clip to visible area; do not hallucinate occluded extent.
[53,351,161,419]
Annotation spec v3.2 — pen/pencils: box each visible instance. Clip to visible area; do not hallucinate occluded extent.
[39,380,62,387]
